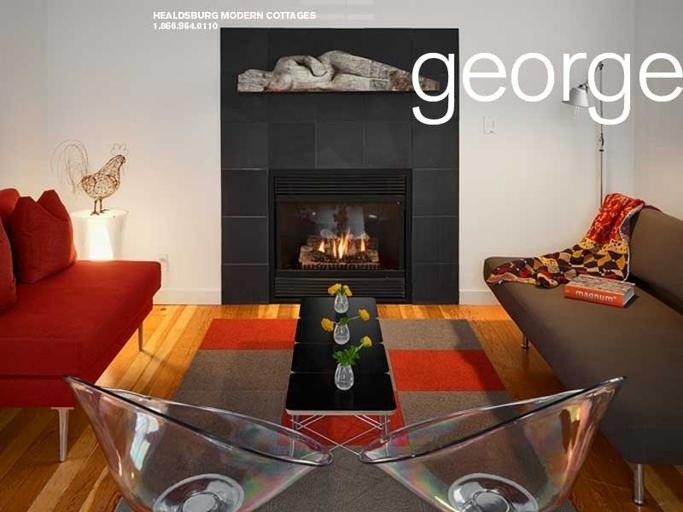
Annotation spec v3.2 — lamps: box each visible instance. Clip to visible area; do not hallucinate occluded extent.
[72,209,126,262]
[559,59,605,207]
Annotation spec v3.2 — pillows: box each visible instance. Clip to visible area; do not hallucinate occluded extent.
[11,189,79,284]
[0,220,18,314]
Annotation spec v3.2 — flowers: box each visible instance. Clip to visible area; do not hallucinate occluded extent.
[327,284,352,298]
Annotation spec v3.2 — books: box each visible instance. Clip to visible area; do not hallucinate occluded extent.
[562,273,638,308]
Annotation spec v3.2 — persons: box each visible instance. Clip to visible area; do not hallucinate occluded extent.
[235,48,442,93]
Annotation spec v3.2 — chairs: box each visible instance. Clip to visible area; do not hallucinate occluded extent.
[61,371,330,511]
[357,373,625,512]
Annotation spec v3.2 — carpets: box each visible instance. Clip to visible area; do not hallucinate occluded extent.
[108,319,577,511]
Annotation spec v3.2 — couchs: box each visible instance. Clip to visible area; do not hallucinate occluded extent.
[0,188,162,462]
[482,193,682,508]
